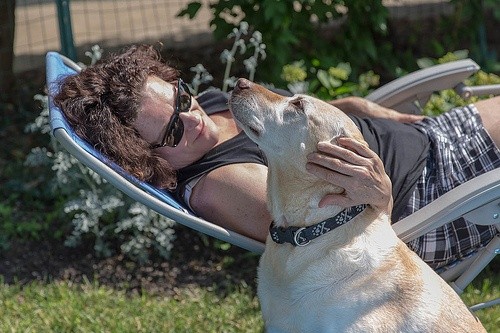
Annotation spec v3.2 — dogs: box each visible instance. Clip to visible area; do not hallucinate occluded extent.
[225,77,489,333]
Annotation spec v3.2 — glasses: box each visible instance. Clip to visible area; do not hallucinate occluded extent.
[142,77,193,151]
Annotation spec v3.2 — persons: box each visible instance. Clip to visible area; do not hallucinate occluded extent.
[53,39,500,271]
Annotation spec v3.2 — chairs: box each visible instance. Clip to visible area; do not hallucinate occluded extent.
[45,49,500,312]
